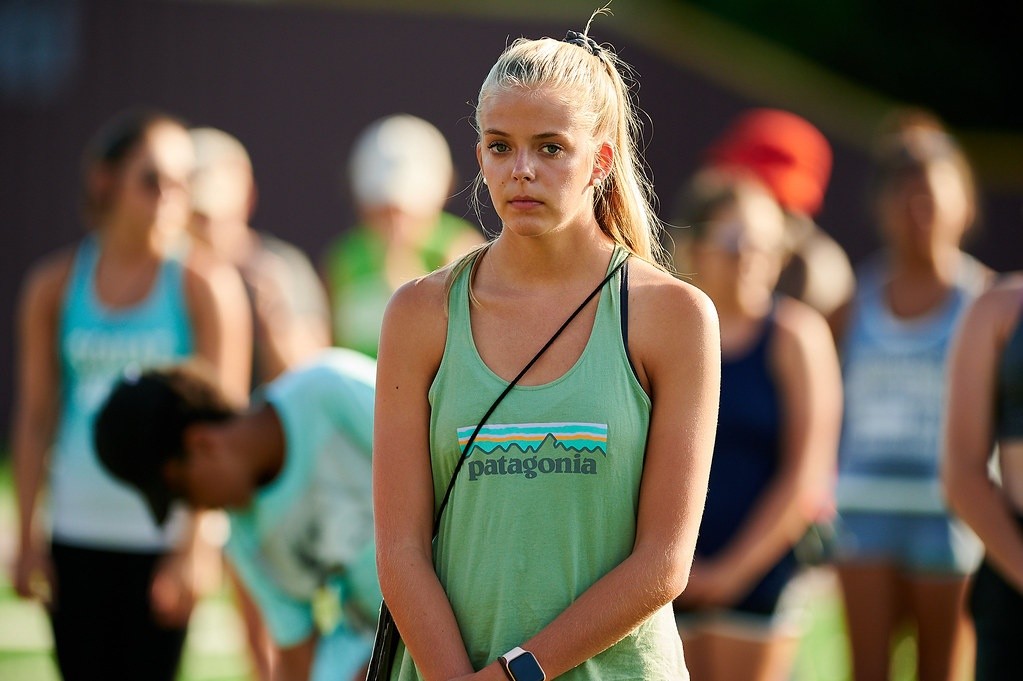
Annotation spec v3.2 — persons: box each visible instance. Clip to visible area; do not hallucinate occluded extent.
[11,113,333,679]
[670,109,1023,681]
[320,112,488,358]
[93,346,378,681]
[372,30,721,681]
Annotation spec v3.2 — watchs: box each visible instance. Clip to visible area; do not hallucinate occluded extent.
[501,646,547,681]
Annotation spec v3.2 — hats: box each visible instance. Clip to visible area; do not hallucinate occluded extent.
[349,113,452,218]
[183,125,255,221]
[93,370,217,529]
[706,107,833,221]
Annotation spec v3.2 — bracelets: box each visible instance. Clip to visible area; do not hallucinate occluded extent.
[498,657,515,681]
